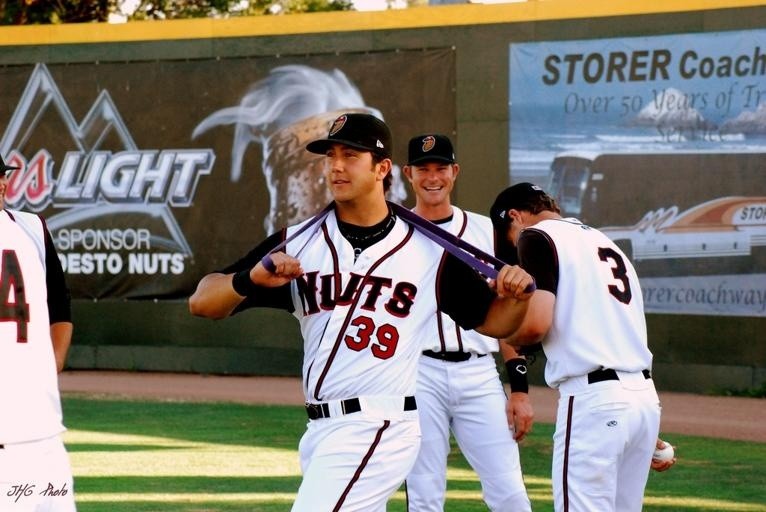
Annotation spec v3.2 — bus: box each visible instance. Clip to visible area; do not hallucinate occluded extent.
[542,147,766,275]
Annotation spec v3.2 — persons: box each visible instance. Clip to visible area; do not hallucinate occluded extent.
[388,135,535,511]
[0,153,77,512]
[189,112,537,512]
[488,180,678,512]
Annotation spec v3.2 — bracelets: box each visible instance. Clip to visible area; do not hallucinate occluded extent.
[506,356,529,393]
[233,267,254,298]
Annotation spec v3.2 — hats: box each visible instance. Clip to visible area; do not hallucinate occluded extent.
[406,134,455,167]
[0,154,22,176]
[306,111,395,159]
[489,183,547,257]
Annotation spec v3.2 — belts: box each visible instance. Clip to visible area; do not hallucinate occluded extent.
[588,361,651,384]
[305,395,419,421]
[422,348,490,362]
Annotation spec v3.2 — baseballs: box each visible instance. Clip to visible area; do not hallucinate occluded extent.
[652,440,675,464]
